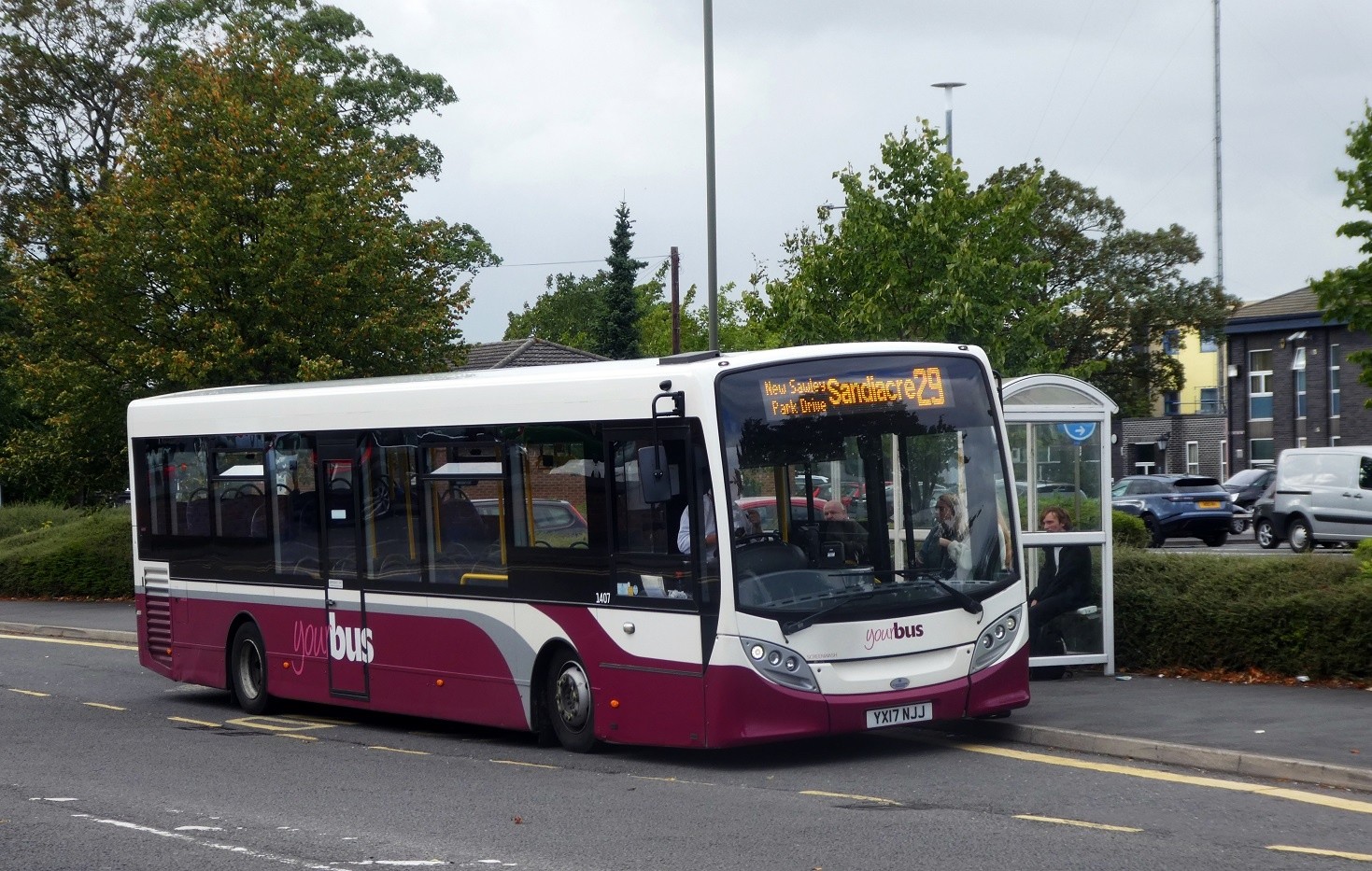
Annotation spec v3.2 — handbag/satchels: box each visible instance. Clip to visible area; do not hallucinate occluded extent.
[1029,628,1072,681]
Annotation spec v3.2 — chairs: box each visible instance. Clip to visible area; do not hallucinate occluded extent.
[184,483,602,585]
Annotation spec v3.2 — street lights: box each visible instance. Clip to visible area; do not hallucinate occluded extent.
[931,80,968,157]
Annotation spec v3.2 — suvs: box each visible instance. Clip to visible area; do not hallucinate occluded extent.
[1112,471,1234,548]
[471,495,588,538]
[1222,464,1284,550]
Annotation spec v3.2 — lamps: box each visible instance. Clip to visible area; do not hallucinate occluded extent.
[1227,364,1242,378]
[1156,432,1170,452]
[1278,338,1285,349]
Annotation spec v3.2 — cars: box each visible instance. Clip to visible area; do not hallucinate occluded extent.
[734,473,1088,520]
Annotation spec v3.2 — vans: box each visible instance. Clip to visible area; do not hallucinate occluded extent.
[1274,445,1372,554]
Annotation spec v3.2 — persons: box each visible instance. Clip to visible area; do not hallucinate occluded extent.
[1026,506,1093,657]
[940,491,1011,582]
[824,499,868,549]
[917,492,959,581]
[677,468,764,561]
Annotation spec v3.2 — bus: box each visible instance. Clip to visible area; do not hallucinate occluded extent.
[125,338,1035,753]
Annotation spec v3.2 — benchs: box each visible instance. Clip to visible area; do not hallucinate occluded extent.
[1051,607,1103,629]
